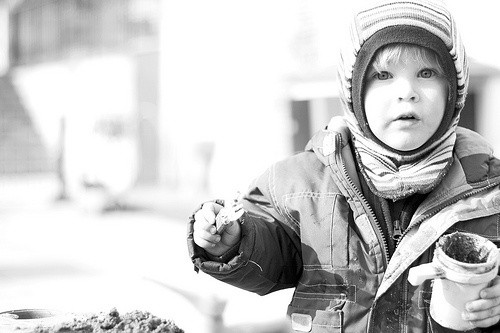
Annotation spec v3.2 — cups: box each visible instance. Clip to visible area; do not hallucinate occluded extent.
[407,232,500,331]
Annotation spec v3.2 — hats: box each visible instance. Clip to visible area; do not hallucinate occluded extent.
[328,0,471,200]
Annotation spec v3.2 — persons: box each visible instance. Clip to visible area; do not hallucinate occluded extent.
[187,0,500,333]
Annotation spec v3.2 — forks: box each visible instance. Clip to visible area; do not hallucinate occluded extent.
[216,196,245,236]
